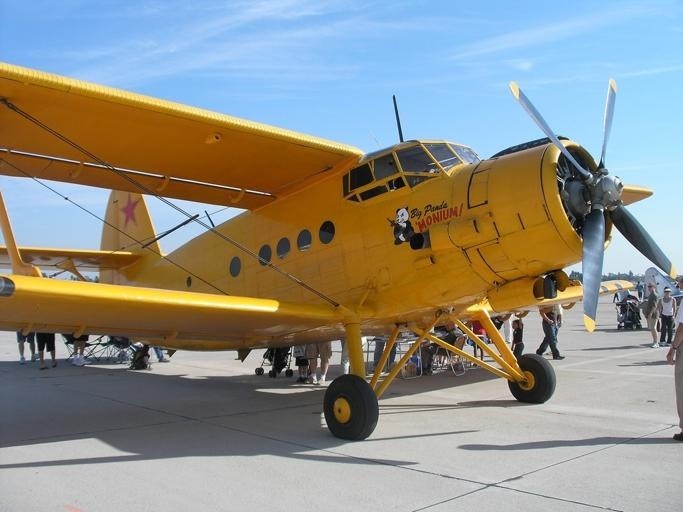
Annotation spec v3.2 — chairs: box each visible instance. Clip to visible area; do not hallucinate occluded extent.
[428,335,465,376]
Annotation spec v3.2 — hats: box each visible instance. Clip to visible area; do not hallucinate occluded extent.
[647,283,655,287]
[664,287,672,292]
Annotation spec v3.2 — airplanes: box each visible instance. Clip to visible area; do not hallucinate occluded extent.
[0,58,678,443]
[614,265,682,331]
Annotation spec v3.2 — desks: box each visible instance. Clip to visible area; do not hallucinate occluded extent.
[366,333,483,380]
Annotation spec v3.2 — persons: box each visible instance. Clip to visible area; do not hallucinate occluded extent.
[15,330,39,364]
[152,345,169,362]
[656,286,677,347]
[535,303,565,360]
[293,344,309,384]
[510,317,524,357]
[665,276,682,440]
[371,333,397,372]
[640,282,660,348]
[414,323,464,377]
[304,340,332,384]
[70,333,91,366]
[635,281,643,299]
[469,320,487,368]
[35,332,56,369]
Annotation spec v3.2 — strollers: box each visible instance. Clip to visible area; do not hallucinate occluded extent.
[614,300,641,331]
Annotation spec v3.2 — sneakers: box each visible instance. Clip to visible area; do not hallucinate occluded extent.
[297,374,325,385]
[674,432,683,441]
[650,342,671,348]
[20,355,92,370]
[159,358,169,362]
[553,356,564,359]
[536,349,542,355]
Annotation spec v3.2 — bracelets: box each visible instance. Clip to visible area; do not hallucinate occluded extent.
[669,344,678,350]
[545,317,550,322]
